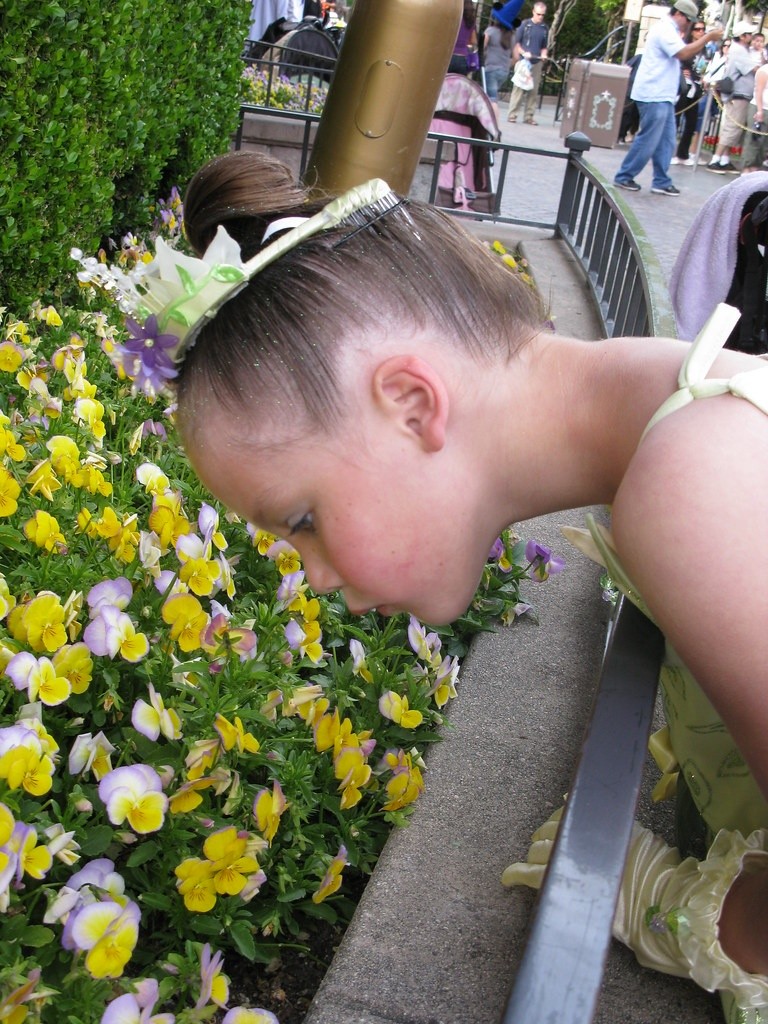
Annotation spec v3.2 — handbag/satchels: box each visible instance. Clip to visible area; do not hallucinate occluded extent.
[468,46,479,71]
[715,77,733,94]
[510,60,534,90]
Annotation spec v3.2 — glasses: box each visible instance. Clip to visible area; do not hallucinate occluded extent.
[691,26,706,32]
[722,44,730,48]
[534,11,545,16]
[679,11,692,22]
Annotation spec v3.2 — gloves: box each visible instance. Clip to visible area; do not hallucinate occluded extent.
[502,794,768,1009]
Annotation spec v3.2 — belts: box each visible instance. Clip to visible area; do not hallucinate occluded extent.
[731,94,748,99]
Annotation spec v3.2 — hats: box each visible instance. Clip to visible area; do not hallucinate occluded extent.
[732,21,757,37]
[673,0,699,18]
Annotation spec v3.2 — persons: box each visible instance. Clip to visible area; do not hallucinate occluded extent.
[612,0,768,197]
[70,150,768,1024]
[446,0,549,126]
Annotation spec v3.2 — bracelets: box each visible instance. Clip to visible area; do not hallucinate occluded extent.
[757,111,763,115]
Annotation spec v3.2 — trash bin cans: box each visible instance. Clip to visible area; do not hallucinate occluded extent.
[561,59,631,148]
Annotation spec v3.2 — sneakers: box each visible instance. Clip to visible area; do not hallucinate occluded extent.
[613,180,641,191]
[705,162,725,174]
[720,163,740,174]
[670,157,695,165]
[651,184,680,195]
[690,154,707,166]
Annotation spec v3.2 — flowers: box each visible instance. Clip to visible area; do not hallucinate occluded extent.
[105,304,181,397]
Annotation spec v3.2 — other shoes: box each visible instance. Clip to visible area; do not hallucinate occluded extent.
[508,118,516,122]
[524,119,538,125]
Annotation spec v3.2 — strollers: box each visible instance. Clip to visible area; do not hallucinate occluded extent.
[428,54,502,221]
